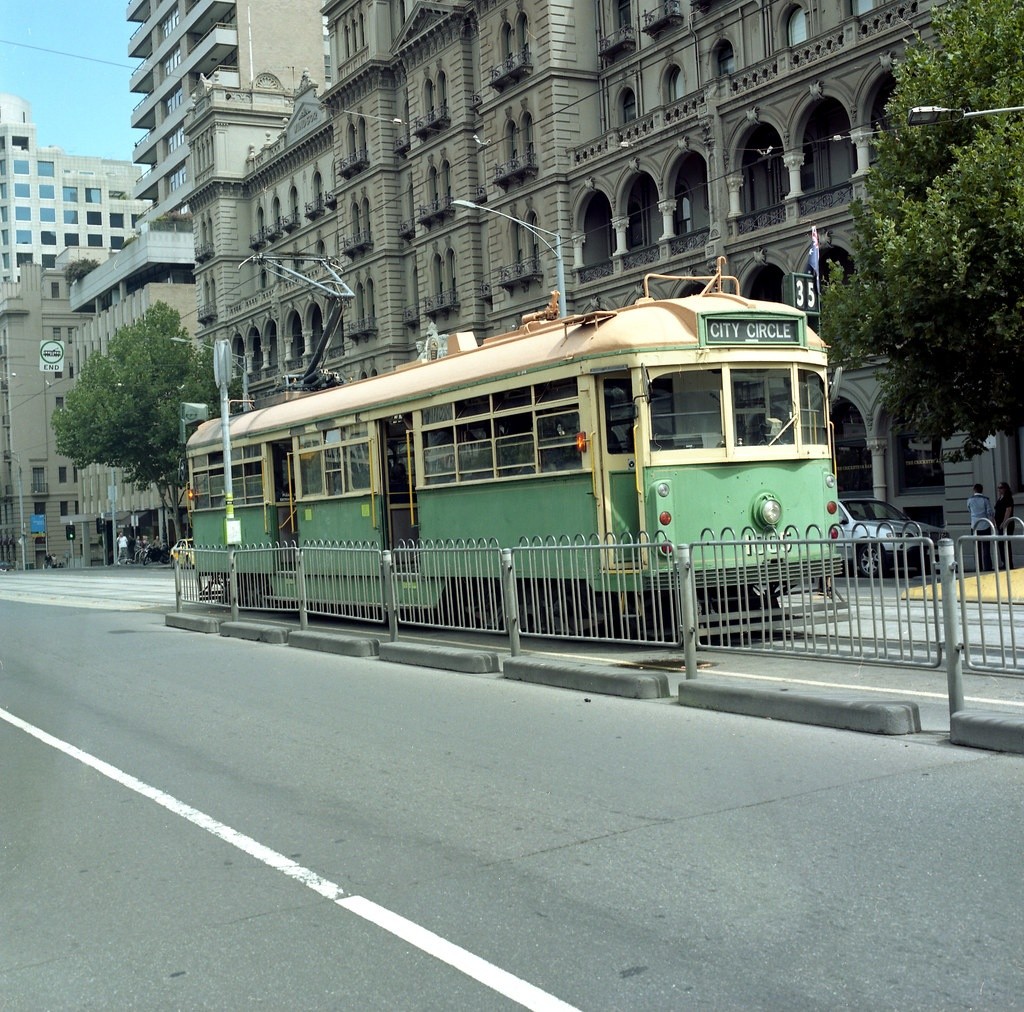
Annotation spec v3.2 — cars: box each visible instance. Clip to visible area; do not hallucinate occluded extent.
[170,539,195,568]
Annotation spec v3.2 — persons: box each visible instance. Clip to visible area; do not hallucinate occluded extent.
[116,531,130,565]
[45,553,57,568]
[966,484,995,572]
[993,481,1014,569]
[134,535,162,551]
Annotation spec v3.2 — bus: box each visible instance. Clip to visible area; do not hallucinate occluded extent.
[186,256,843,643]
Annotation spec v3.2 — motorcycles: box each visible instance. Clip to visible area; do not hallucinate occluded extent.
[143,544,169,565]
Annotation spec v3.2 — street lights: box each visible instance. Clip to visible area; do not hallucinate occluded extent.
[170,337,249,413]
[452,200,567,322]
[158,481,166,544]
[4,451,26,571]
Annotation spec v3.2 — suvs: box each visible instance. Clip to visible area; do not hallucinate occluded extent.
[838,498,950,578]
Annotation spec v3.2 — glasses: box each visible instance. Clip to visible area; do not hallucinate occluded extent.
[998,486,1003,489]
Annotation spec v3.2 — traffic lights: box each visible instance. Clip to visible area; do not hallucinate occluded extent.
[66,525,75,541]
[96,518,102,534]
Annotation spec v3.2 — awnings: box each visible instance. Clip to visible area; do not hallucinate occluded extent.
[116,511,152,527]
